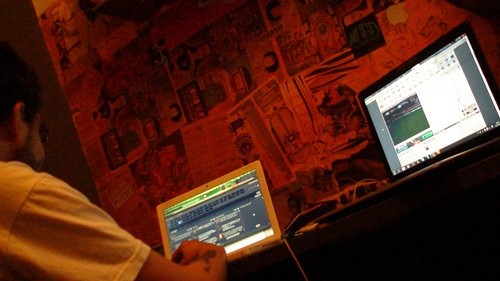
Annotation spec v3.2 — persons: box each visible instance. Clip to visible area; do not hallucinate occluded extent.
[0,41,228,281]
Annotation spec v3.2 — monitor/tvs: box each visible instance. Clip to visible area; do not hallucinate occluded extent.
[358,21,500,183]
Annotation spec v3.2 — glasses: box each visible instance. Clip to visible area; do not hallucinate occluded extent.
[26,104,49,144]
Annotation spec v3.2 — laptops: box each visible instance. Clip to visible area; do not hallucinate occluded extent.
[156,160,282,263]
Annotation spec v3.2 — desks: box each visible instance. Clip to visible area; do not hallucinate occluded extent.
[224,149,500,281]
